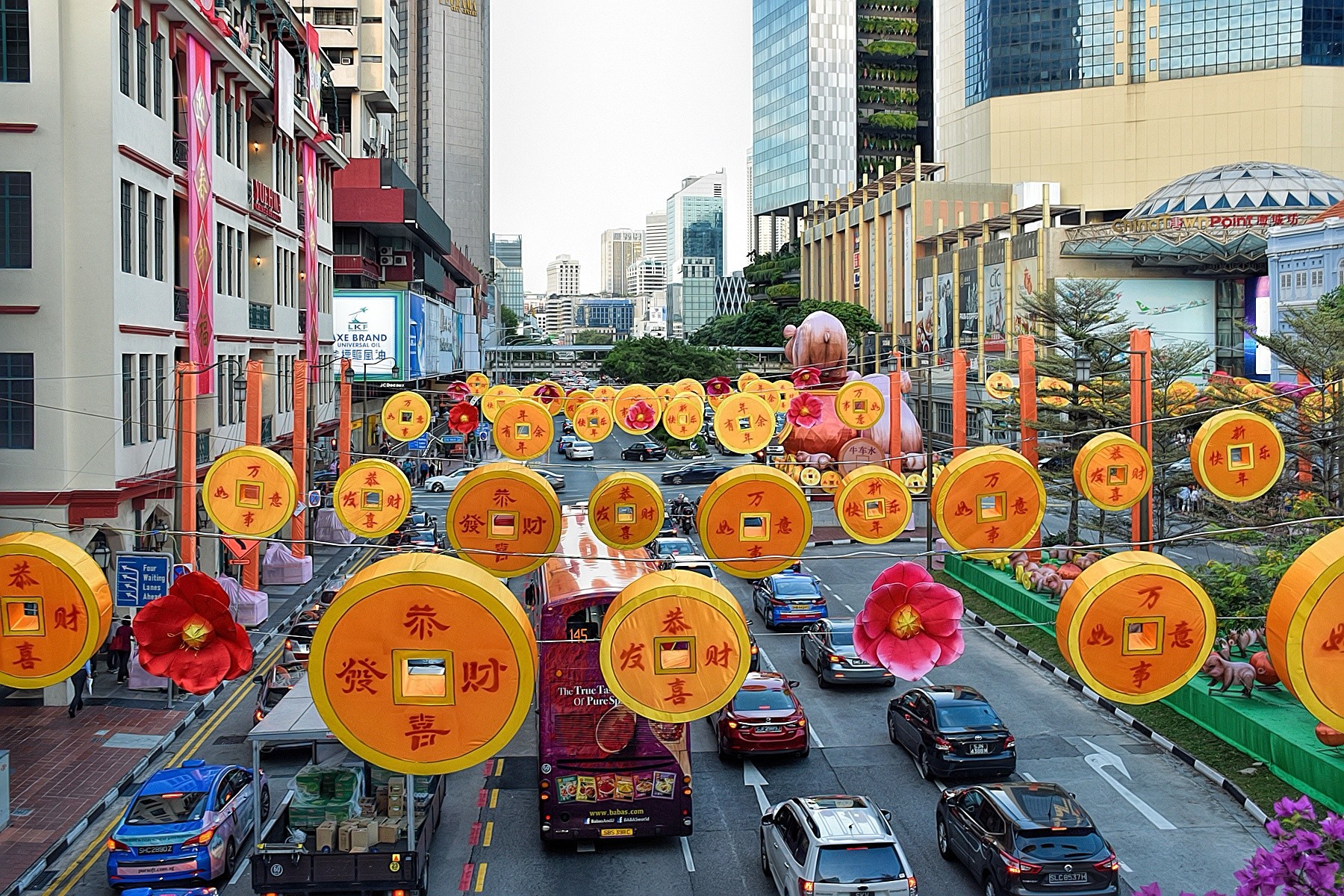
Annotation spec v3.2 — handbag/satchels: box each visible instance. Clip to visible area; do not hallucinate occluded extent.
[110,636,124,650]
[86,677,93,695]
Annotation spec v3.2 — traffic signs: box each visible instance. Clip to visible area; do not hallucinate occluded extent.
[114,554,170,608]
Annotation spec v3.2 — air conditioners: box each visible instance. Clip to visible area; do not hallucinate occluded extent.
[380,246,393,256]
[394,255,406,266]
[380,256,393,265]
[342,58,351,64]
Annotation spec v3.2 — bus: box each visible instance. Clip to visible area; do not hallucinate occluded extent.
[247,671,446,896]
[522,500,695,855]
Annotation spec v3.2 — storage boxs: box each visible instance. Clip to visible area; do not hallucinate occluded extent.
[289,762,434,852]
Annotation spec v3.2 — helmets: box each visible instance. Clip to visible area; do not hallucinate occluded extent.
[678,493,684,499]
[683,497,688,503]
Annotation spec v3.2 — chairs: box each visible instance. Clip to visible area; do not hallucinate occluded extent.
[680,547,688,551]
[568,623,599,641]
[661,548,670,552]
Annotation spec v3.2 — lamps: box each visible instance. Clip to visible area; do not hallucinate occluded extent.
[254,140,258,152]
[256,255,261,266]
[300,271,304,279]
[299,174,303,183]
[133,518,170,552]
[196,501,210,531]
[91,541,112,570]
[314,444,327,463]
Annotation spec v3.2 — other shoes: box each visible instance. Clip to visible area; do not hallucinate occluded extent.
[77,705,83,710]
[68,708,75,718]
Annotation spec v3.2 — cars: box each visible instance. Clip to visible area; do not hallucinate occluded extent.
[620,440,668,462]
[644,502,719,582]
[758,793,920,896]
[800,617,896,689]
[421,467,476,493]
[706,671,810,764]
[696,401,785,464]
[530,468,566,491]
[660,460,735,486]
[105,758,272,891]
[936,781,1121,896]
[253,471,447,755]
[752,570,829,630]
[887,685,1017,783]
[556,406,594,461]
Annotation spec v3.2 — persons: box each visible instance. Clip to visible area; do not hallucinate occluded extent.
[350,441,354,452]
[1015,447,1020,453]
[89,612,137,683]
[437,460,443,476]
[677,497,698,534]
[395,458,417,486]
[669,493,684,511]
[329,459,336,473]
[430,450,435,463]
[68,660,91,718]
[420,460,428,486]
[374,424,380,445]
[434,407,449,418]
[938,279,954,347]
[379,441,388,454]
[428,460,436,477]
[1169,485,1201,512]
[314,484,327,519]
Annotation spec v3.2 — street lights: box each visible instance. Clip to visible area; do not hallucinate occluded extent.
[867,331,907,372]
[362,357,399,461]
[305,358,356,579]
[886,343,933,572]
[172,359,248,565]
[1073,336,1150,553]
[480,325,542,387]
